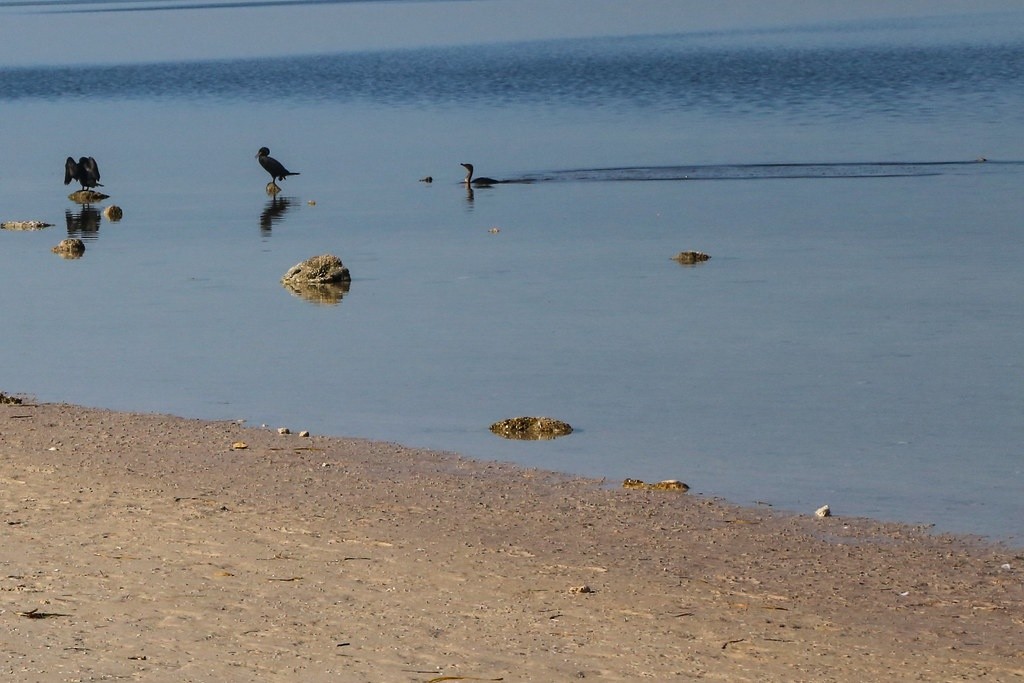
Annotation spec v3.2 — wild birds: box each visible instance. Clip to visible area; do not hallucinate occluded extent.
[461,163,500,184]
[64,156,104,191]
[255,146,301,186]
